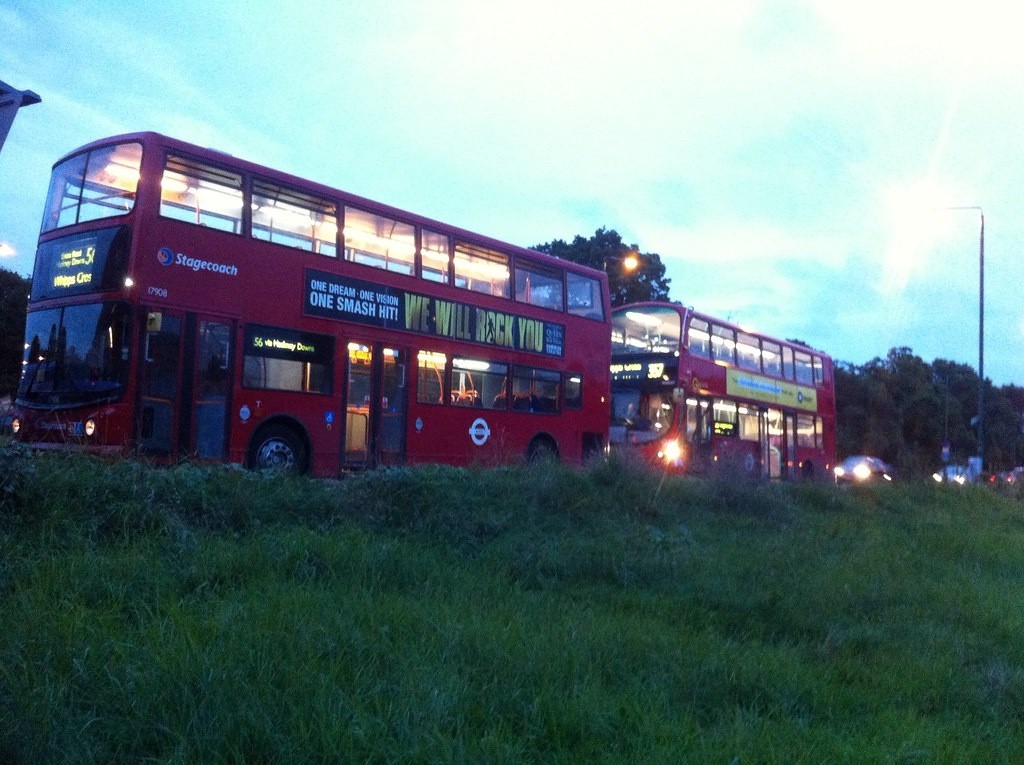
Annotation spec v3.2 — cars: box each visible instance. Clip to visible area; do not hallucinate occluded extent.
[833,456,893,484]
[931,466,974,484]
[990,471,1015,482]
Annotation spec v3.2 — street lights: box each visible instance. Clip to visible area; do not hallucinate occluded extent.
[965,204,985,459]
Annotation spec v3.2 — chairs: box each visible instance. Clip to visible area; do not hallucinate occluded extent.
[768,432,824,447]
[688,342,823,383]
[436,389,575,413]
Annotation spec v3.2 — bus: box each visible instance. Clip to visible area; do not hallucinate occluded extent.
[12,130,611,489]
[607,302,837,488]
